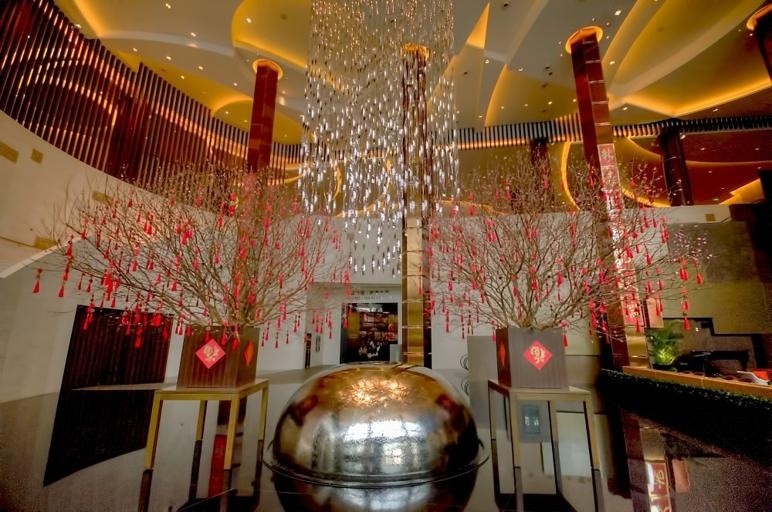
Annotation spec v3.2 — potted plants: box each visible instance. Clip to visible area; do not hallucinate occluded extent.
[645,320,689,369]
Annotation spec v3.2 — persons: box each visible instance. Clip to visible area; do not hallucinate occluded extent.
[735,369,772,385]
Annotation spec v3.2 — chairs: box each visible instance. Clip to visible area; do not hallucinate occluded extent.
[679,351,705,371]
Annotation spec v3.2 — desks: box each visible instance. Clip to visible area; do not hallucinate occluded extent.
[490,439,606,512]
[143,377,271,470]
[137,441,264,512]
[487,379,600,470]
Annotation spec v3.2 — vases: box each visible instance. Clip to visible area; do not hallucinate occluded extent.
[495,327,569,390]
[176,324,260,389]
